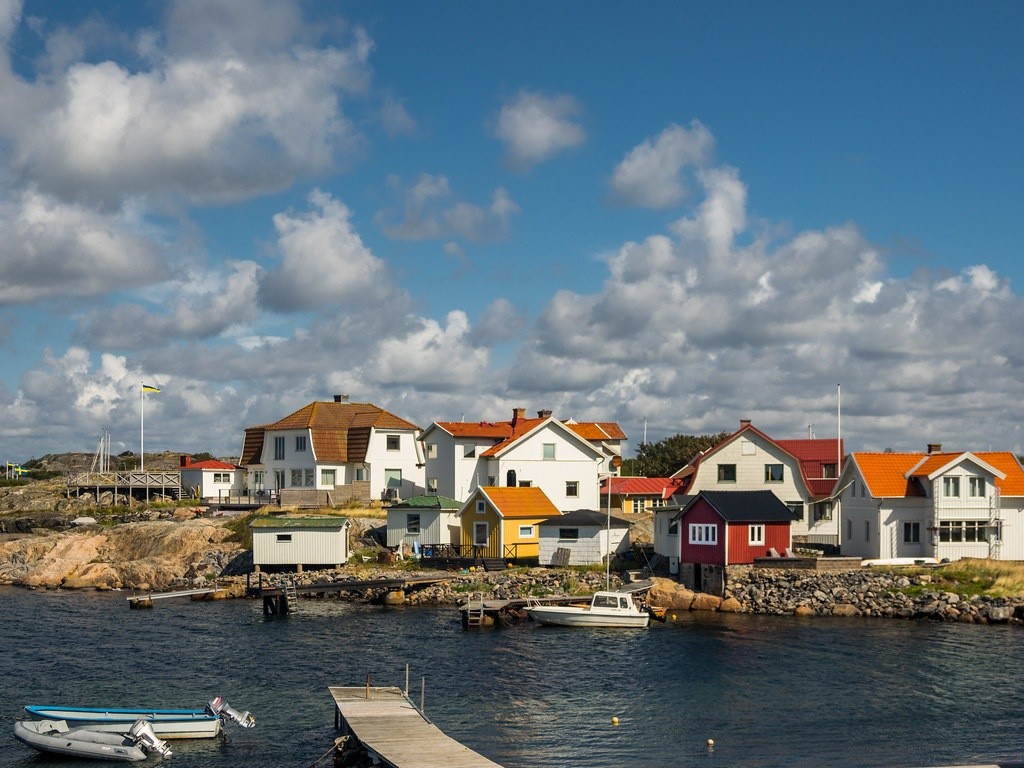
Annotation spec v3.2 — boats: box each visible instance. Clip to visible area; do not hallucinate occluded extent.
[25,696,255,744]
[13,718,173,762]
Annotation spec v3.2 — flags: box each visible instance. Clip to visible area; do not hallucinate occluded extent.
[8,463,28,475]
[143,385,160,394]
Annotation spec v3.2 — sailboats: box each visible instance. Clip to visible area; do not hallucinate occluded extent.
[522,476,652,629]
